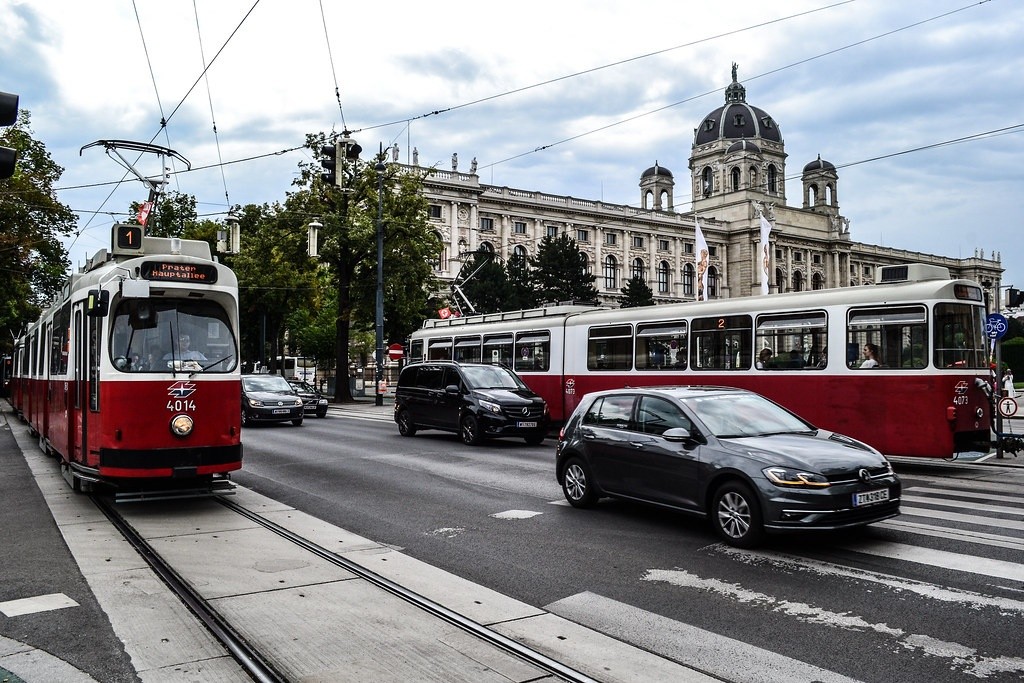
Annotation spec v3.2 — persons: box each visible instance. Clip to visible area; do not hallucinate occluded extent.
[674,351,686,369]
[163,330,207,360]
[859,344,878,368]
[760,348,773,369]
[1002,369,1015,398]
[130,345,161,371]
[903,344,924,368]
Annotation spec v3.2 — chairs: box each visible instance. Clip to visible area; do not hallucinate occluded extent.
[787,349,800,368]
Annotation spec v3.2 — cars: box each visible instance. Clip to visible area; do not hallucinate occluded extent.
[554,384,903,550]
[240,373,305,428]
[286,379,329,418]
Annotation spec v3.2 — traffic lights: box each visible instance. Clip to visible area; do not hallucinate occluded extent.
[321,143,345,189]
[4,357,13,379]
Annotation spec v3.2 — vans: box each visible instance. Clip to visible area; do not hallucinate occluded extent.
[393,359,553,447]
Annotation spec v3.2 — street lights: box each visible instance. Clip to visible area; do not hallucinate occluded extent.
[372,142,389,406]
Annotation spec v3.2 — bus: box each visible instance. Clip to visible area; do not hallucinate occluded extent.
[241,355,316,388]
[0,235,244,503]
[402,261,996,460]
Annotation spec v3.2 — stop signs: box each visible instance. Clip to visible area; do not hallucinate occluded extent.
[387,344,403,361]
